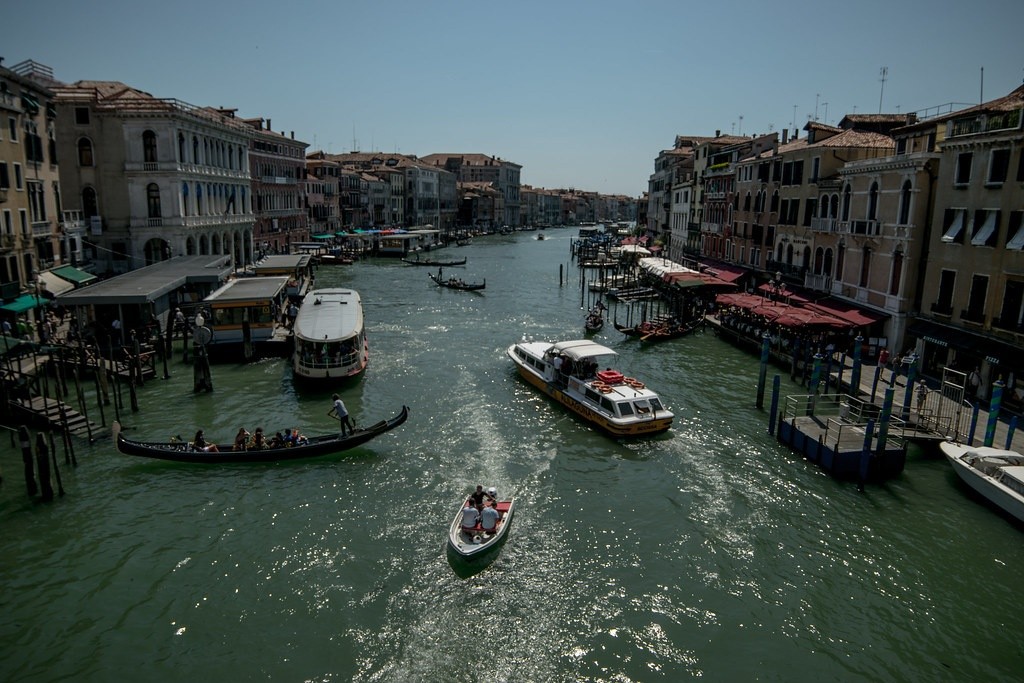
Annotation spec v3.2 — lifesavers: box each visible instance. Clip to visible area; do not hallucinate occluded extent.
[591,380,613,393]
[624,377,645,388]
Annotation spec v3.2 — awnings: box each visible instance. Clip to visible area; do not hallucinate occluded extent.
[639,235,662,252]
[698,259,889,326]
[311,227,407,238]
[0,263,97,314]
[907,318,1024,370]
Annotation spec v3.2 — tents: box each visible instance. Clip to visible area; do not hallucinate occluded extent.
[638,256,850,362]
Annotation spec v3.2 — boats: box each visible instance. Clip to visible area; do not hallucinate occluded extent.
[290,288,369,385]
[456,238,472,246]
[117,405,411,463]
[938,436,1024,524]
[427,272,486,290]
[448,487,515,557]
[401,256,467,266]
[506,340,675,436]
[573,218,705,343]
[537,234,545,240]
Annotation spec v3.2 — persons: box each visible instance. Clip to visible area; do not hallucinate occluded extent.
[553,354,598,389]
[482,501,498,535]
[192,427,310,452]
[271,299,356,367]
[438,266,465,287]
[968,366,984,405]
[2,306,229,345]
[327,241,420,262]
[716,305,916,383]
[586,293,714,336]
[463,499,482,529]
[471,485,495,514]
[327,393,354,439]
[914,379,928,414]
